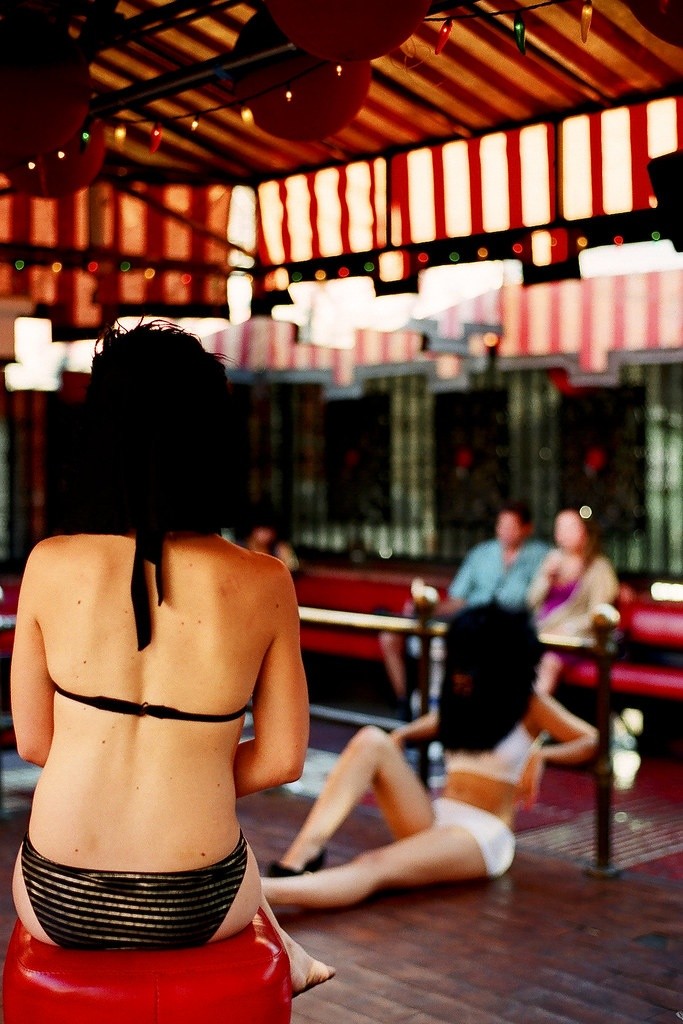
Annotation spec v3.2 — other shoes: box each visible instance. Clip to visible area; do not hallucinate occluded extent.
[267,847,326,878]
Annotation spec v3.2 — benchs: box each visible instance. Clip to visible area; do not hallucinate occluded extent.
[292,563,683,761]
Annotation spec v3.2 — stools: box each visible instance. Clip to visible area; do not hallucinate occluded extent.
[2,906,291,1024]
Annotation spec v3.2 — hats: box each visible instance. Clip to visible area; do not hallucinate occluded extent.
[437,603,544,751]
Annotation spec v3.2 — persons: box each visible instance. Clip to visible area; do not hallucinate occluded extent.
[245,505,617,906]
[11,316,337,997]
